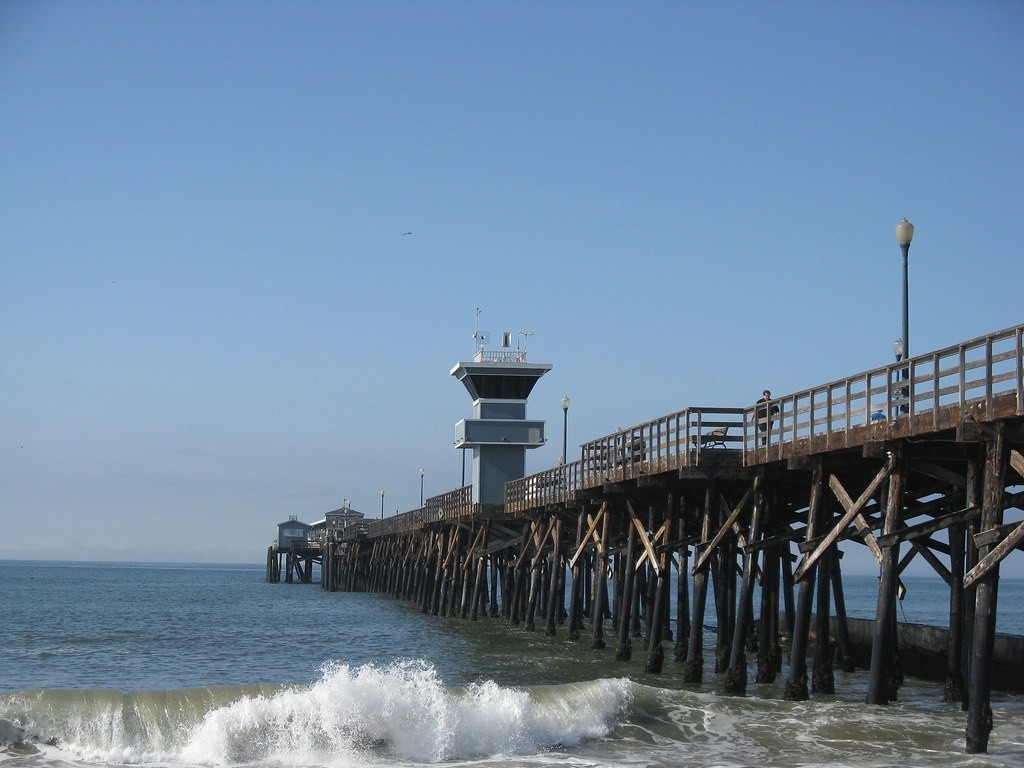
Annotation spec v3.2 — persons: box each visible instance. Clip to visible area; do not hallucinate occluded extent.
[872,409,887,421]
[750,389,779,445]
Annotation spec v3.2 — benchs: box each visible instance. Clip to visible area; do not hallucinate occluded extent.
[692,426,729,449]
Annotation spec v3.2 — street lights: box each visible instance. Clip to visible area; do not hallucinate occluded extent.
[559,395,571,465]
[381,490,385,518]
[395,506,399,515]
[419,468,426,507]
[891,217,915,415]
